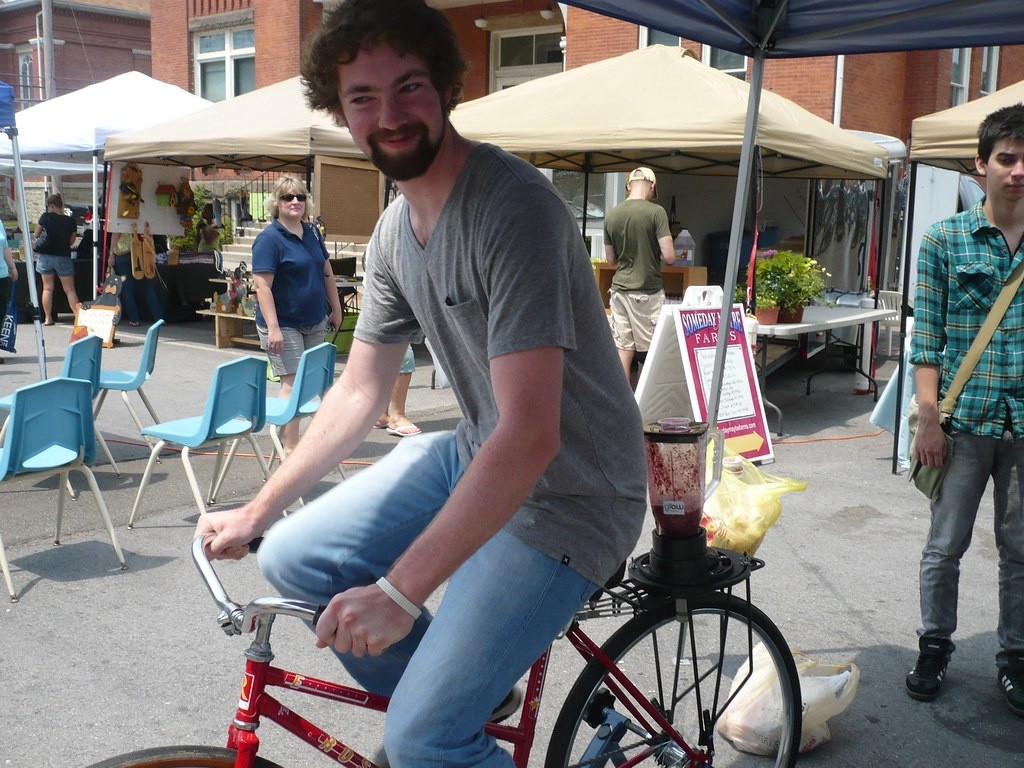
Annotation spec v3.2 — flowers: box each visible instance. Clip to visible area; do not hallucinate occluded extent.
[729,248,835,310]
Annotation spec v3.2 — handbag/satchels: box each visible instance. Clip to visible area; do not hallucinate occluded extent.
[719,640,860,756]
[32,213,47,250]
[702,443,808,556]
[0,281,18,353]
[905,396,952,498]
[114,233,131,255]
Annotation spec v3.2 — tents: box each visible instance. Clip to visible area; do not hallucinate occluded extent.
[97,75,391,296]
[892,80,1024,470]
[448,42,892,393]
[0,160,110,213]
[559,0,1024,430]
[0,71,214,380]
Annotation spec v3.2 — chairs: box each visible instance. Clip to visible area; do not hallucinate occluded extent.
[263,341,348,507]
[0,377,128,605]
[876,290,915,356]
[0,334,123,499]
[94,318,173,464]
[129,356,289,529]
[328,257,359,317]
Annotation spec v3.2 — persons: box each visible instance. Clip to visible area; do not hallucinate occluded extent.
[604,166,675,383]
[34,193,79,326]
[195,203,224,271]
[0,219,18,363]
[906,104,1024,716]
[374,343,422,436]
[107,233,167,326]
[252,174,343,464]
[193,0,648,768]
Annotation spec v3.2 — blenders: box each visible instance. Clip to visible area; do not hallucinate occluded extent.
[627,417,750,591]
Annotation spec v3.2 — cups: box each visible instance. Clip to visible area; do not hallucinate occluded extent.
[11,248,21,261]
[168,250,178,265]
[19,239,27,260]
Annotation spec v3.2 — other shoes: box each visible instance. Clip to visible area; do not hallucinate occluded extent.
[129,321,138,327]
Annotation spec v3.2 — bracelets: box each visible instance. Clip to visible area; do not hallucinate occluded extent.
[109,253,116,256]
[376,576,422,619]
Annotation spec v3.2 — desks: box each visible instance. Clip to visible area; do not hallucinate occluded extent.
[6,258,100,318]
[149,260,225,323]
[197,307,260,347]
[757,306,897,437]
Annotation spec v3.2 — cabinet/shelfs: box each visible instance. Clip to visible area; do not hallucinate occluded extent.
[593,264,707,315]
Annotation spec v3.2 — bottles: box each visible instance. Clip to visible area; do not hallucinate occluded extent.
[671,230,695,265]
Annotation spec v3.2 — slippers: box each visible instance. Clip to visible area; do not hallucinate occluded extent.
[45,321,55,325]
[373,419,388,429]
[386,424,422,436]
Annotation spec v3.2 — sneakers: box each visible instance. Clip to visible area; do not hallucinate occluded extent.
[997,664,1024,713]
[907,635,956,696]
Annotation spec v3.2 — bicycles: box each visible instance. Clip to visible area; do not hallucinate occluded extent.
[69,532,803,767]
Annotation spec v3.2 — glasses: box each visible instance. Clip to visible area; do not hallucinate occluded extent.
[280,194,307,201]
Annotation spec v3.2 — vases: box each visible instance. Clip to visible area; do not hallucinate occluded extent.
[777,302,805,323]
[755,306,780,324]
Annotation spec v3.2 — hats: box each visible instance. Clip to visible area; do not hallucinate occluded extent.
[629,166,658,199]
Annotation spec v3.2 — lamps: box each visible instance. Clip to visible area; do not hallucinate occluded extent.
[539,3,554,20]
[474,1,488,29]
